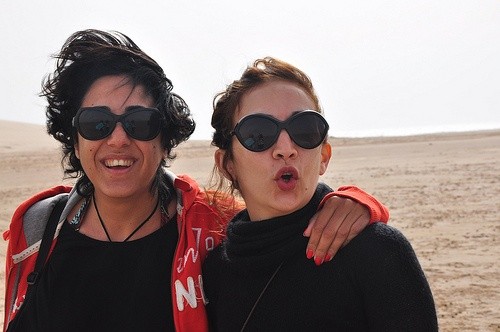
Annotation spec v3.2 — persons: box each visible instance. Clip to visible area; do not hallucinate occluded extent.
[3,28,389,332]
[200,56,439,332]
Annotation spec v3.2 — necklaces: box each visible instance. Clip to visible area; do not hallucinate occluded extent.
[239,256,286,332]
[92,192,159,242]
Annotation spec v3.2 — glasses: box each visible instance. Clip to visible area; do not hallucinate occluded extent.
[71,107,165,142]
[224,110,331,153]
